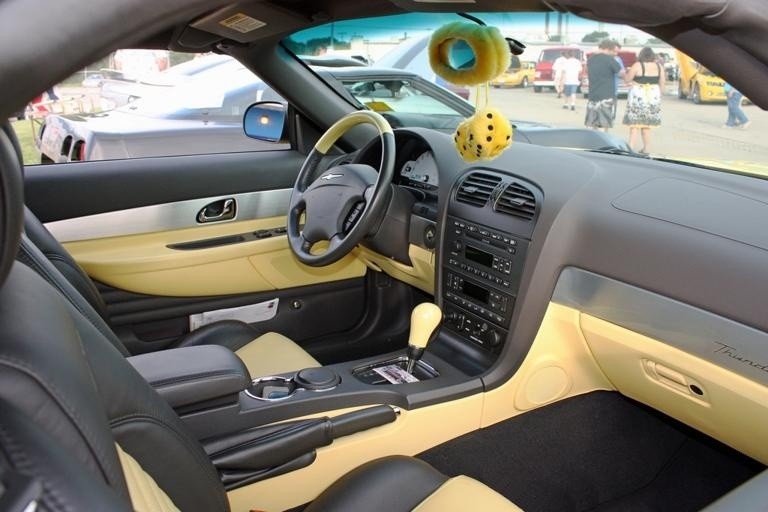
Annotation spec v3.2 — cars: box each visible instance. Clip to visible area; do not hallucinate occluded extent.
[38,65,641,165]
[104,51,373,105]
[487,44,758,106]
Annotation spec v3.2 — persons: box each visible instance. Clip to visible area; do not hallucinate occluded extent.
[559,50,583,111]
[622,47,666,154]
[584,39,627,135]
[551,52,567,100]
[656,53,665,66]
[609,40,627,122]
[720,82,752,130]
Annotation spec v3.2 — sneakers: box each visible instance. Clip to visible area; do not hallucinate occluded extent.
[563,104,574,110]
[721,120,752,129]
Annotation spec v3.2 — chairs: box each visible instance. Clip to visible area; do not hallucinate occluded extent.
[0,116,325,380]
[0,127,528,512]
